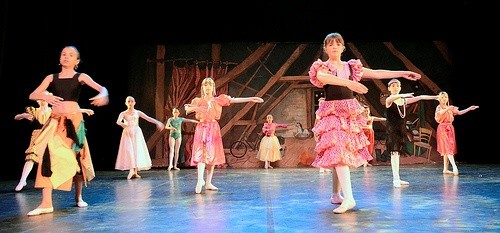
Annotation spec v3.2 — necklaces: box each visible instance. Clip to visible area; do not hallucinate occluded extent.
[395,100,406,118]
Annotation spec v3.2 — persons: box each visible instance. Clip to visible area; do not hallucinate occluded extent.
[259,114,290,169]
[363,103,388,167]
[434,90,480,176]
[312,96,333,174]
[116,95,164,179]
[163,104,200,170]
[184,76,264,194]
[26,44,109,216]
[309,32,421,215]
[15,91,95,191]
[383,77,442,184]
